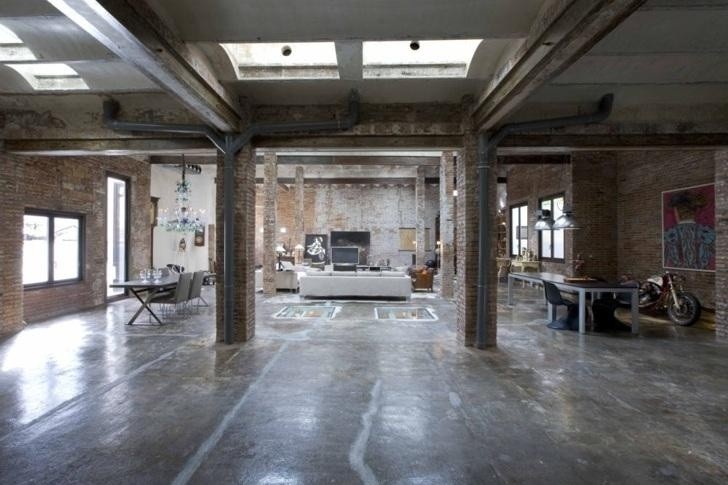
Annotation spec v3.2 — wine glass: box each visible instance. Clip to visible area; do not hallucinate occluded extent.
[140,269,162,284]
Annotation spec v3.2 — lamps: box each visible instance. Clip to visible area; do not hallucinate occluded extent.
[155,154,207,233]
[534,155,583,231]
[276,247,286,270]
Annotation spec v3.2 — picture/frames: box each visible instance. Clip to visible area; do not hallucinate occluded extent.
[661,182,714,273]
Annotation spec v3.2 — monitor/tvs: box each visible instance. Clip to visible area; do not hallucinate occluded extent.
[331,245,360,266]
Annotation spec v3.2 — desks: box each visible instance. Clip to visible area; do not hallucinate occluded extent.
[507,272,639,335]
[108,274,214,325]
[510,261,541,289]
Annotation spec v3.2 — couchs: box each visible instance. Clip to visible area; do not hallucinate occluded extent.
[297,271,412,301]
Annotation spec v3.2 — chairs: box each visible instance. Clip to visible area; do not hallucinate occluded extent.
[542,279,641,333]
[410,265,434,293]
[275,261,321,293]
[145,270,205,326]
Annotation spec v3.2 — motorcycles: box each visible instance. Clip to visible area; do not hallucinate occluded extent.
[614,271,700,326]
